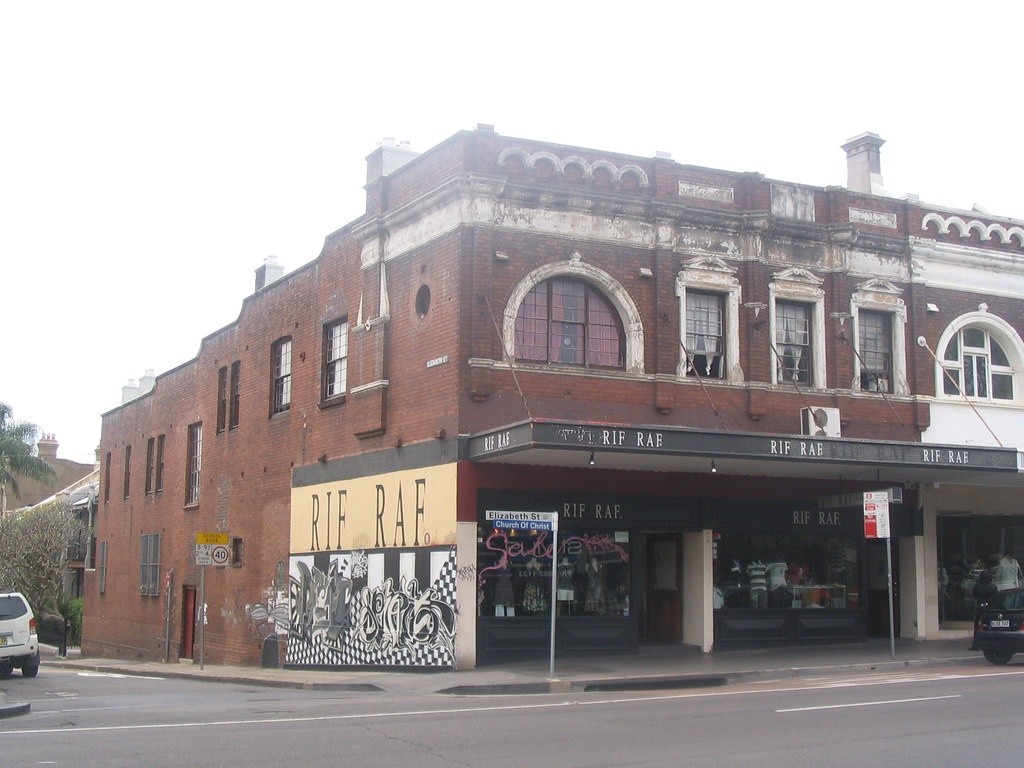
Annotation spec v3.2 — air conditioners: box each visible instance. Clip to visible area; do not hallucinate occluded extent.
[800,406,842,438]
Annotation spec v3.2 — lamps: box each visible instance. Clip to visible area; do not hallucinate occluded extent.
[926,303,940,313]
[589,452,595,466]
[639,267,653,279]
[710,461,718,473]
[494,251,509,261]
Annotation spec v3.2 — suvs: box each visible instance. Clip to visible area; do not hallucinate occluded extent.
[0,593,41,678]
[978,587,1024,666]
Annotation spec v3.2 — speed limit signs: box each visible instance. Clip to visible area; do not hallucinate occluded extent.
[211,546,229,564]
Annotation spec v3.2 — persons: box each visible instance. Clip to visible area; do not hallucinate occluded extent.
[937,548,1022,604]
[720,555,847,609]
[494,554,607,618]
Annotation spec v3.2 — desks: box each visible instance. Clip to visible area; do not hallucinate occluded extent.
[785,584,846,608]
